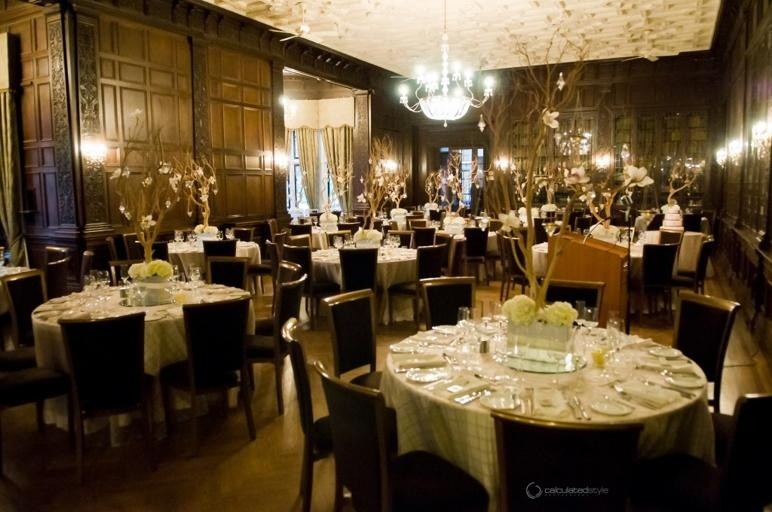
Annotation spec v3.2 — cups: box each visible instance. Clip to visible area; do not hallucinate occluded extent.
[382,205,489,232]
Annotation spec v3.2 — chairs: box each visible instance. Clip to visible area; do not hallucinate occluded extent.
[0,193,772,512]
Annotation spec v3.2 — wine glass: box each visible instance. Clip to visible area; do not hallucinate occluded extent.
[313,218,318,229]
[335,232,402,258]
[78,265,202,318]
[170,229,225,252]
[452,298,626,392]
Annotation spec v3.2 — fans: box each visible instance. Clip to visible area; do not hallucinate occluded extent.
[267,1,343,43]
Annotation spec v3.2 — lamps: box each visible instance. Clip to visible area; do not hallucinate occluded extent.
[392,1,498,130]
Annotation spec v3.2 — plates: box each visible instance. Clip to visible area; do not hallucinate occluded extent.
[389,342,419,354]
[404,368,441,386]
[205,295,235,303]
[648,348,684,358]
[49,293,84,320]
[478,390,521,412]
[664,374,705,391]
[431,324,454,338]
[586,398,634,418]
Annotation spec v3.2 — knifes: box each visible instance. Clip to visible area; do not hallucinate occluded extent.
[573,395,591,421]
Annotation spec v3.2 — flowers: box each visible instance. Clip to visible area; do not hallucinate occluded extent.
[110,26,706,324]
